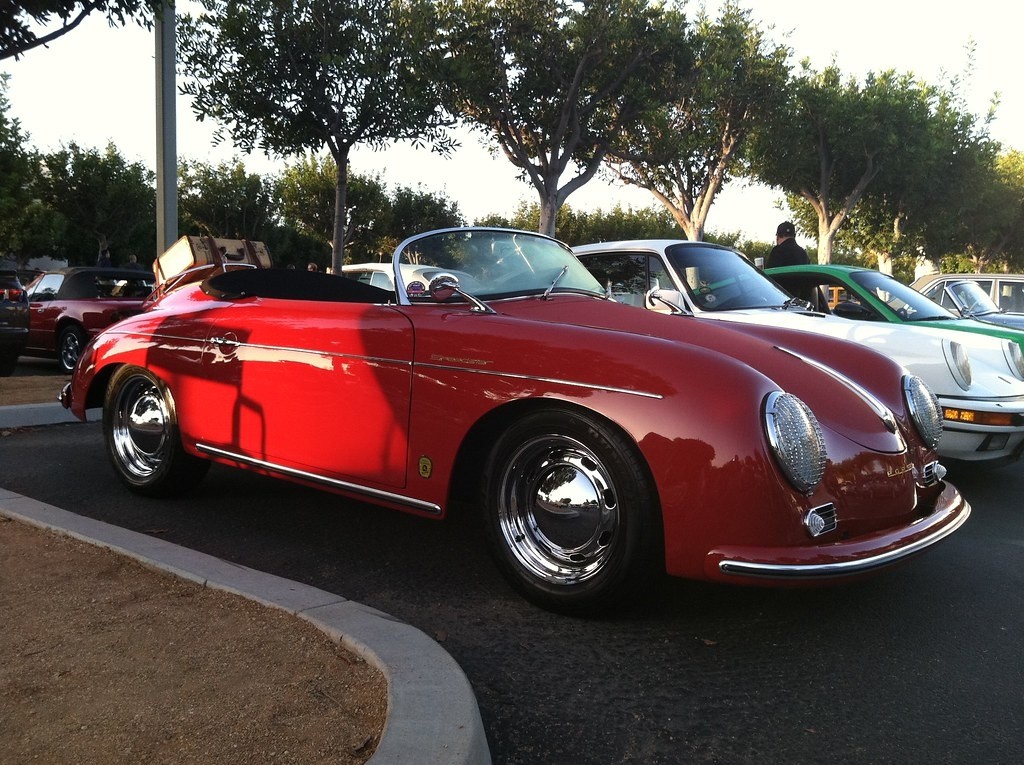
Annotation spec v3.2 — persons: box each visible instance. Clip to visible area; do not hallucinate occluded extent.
[122,255,142,285]
[24,263,40,271]
[767,221,812,300]
[286,263,296,269]
[97,249,111,284]
[307,263,316,272]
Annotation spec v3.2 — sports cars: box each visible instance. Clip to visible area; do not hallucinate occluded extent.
[63,224,973,617]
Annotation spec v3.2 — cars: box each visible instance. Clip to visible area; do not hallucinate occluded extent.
[325,261,486,300]
[688,263,1023,357]
[487,239,1023,465]
[0,266,29,378]
[14,269,164,374]
[910,280,1024,332]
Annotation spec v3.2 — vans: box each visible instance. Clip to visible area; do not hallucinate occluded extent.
[880,274,1023,324]
